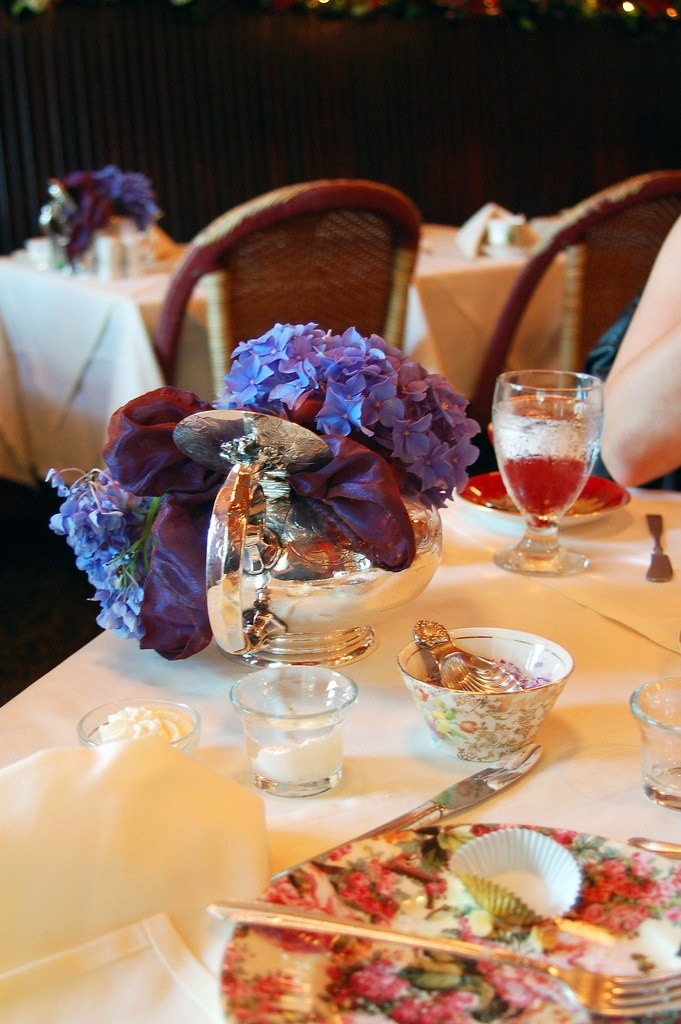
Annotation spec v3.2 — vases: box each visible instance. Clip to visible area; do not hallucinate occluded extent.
[221,478,436,654]
[66,219,145,290]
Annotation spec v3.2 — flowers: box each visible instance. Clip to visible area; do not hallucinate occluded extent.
[46,321,480,661]
[41,160,151,266]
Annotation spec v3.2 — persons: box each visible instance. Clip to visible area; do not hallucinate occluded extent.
[572,210,681,494]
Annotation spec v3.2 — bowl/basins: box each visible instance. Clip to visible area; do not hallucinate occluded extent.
[397,628,575,762]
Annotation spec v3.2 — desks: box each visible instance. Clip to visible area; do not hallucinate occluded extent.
[1,467,681,1024]
[0,218,570,488]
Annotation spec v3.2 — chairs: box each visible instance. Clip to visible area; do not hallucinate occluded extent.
[149,181,424,406]
[469,169,680,435]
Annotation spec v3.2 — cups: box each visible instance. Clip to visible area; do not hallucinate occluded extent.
[630,676,681,812]
[77,699,200,757]
[230,666,358,798]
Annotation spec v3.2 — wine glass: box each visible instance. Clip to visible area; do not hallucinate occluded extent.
[491,370,604,576]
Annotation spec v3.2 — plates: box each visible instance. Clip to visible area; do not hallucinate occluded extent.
[221,824,681,1024]
[459,471,631,524]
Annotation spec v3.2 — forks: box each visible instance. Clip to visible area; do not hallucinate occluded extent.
[207,899,680,1015]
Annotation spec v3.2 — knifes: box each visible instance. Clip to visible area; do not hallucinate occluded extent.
[270,743,544,881]
